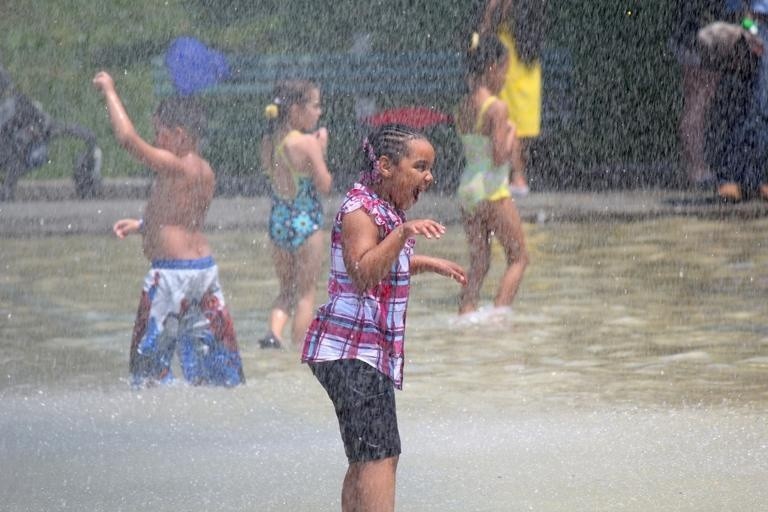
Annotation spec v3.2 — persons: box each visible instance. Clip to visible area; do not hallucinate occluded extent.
[256,78,333,354]
[92,70,244,389]
[300,122,468,512]
[81,137,104,198]
[469,0,544,195]
[667,0,768,201]
[459,35,532,317]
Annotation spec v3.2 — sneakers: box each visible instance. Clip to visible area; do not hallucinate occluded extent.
[705,183,767,205]
